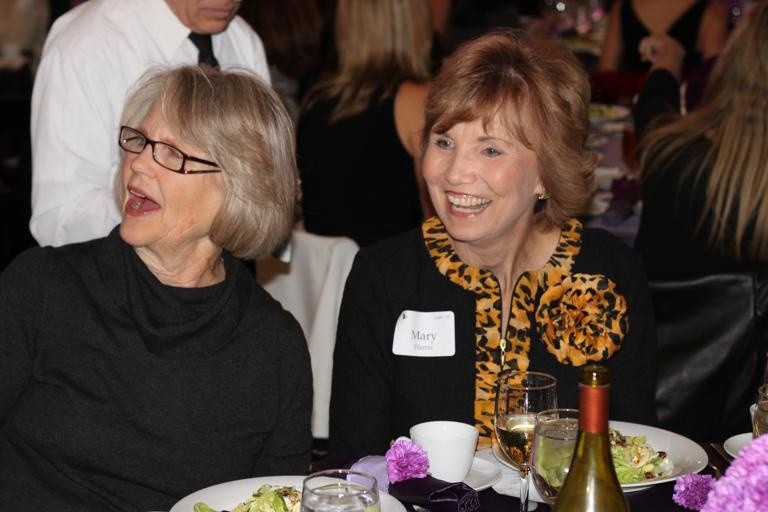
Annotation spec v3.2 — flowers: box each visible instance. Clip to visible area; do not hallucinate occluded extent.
[673,432,768,511]
[384,437,429,484]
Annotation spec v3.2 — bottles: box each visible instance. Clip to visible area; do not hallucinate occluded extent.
[554,365,631,512]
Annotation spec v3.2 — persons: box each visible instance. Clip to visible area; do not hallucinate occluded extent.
[1,62,315,511]
[2,1,723,248]
[330,31,658,475]
[638,6,767,279]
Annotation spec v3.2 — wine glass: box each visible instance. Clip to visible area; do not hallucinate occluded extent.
[494,372,558,512]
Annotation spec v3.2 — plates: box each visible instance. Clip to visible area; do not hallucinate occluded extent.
[464,458,501,493]
[723,432,752,459]
[170,475,407,512]
[608,419,708,493]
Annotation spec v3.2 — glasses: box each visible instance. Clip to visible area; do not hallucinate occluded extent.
[118,126,221,175]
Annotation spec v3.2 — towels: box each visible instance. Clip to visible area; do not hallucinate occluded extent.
[387,476,481,512]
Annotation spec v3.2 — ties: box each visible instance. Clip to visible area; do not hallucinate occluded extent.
[189,31,219,69]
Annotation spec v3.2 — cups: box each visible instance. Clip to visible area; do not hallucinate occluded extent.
[302,469,379,511]
[530,408,580,511]
[410,419,479,482]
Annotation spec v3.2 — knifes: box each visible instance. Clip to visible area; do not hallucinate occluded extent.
[711,442,733,464]
